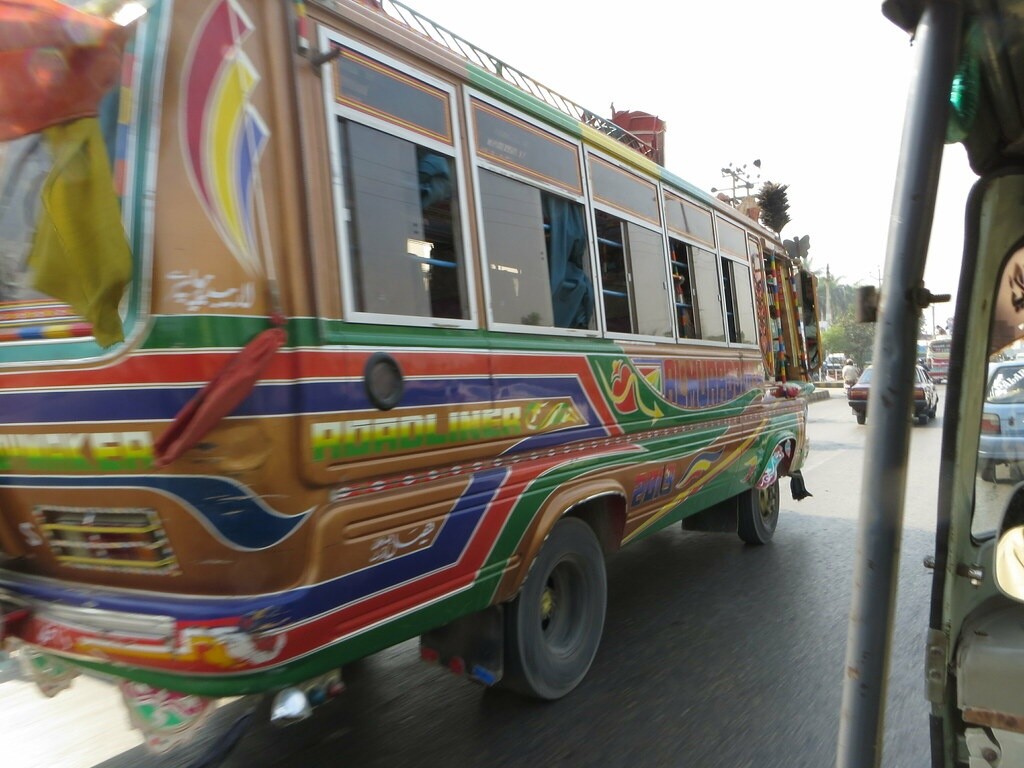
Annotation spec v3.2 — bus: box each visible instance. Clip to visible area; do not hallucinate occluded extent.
[925,335,952,383]
[0,1,829,757]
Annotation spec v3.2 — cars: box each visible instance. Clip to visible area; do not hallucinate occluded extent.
[847,363,939,425]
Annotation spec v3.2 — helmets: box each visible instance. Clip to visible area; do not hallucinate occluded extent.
[846,359,853,365]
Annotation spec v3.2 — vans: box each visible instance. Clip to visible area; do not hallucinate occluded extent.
[978,361,1023,481]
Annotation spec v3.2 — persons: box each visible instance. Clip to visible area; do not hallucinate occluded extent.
[842,358,861,386]
